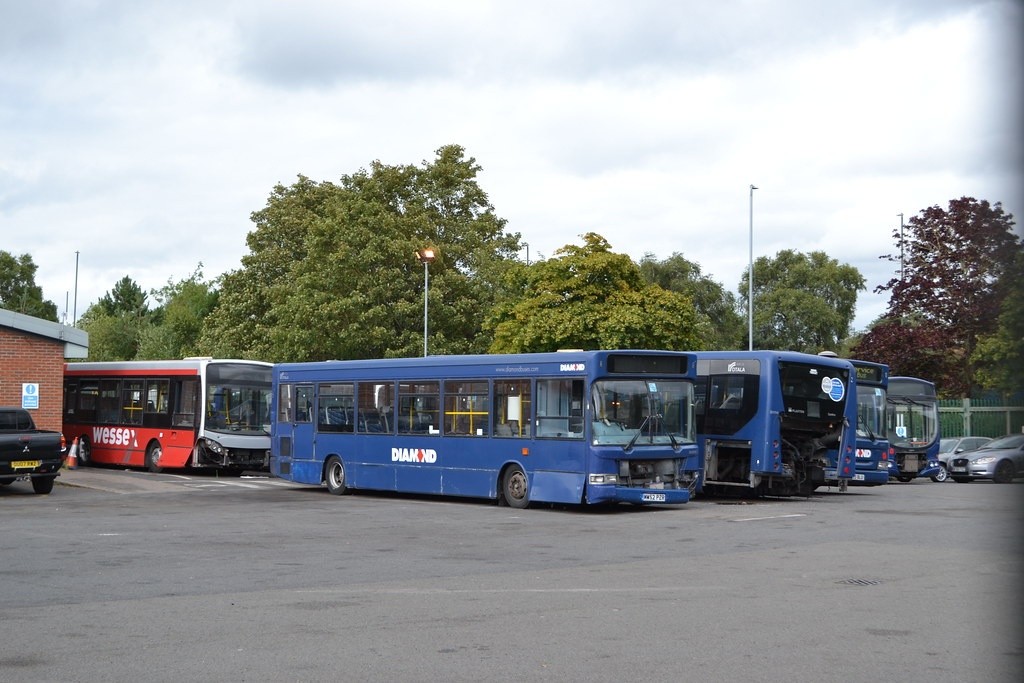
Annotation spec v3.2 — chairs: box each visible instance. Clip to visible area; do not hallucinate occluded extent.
[496,424,513,437]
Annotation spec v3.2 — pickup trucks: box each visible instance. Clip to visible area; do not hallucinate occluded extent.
[0,406,70,492]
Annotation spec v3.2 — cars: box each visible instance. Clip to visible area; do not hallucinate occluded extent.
[946,431,1024,486]
[934,437,995,483]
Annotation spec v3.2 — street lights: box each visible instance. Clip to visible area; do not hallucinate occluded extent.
[899,210,930,288]
[415,249,444,360]
[748,182,772,361]
[76,250,81,334]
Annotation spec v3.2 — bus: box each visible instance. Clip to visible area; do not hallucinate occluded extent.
[885,376,944,481]
[841,359,891,485]
[269,349,703,506]
[64,358,278,473]
[688,350,857,499]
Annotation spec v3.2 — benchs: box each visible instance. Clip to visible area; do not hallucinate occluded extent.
[123,399,155,422]
[308,407,432,433]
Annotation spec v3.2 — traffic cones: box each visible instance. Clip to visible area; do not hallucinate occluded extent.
[63,435,81,471]
